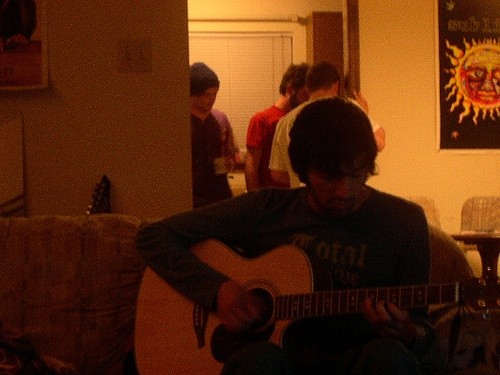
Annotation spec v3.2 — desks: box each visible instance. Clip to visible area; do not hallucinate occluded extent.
[450,234,500,286]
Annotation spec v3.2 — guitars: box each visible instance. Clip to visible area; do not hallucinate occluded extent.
[134,238,500,375]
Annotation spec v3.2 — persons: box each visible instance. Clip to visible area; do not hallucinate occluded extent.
[267,60,387,190]
[244,60,311,192]
[130,94,438,375]
[187,60,242,207]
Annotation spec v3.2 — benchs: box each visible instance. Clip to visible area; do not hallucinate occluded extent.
[0,214,500,375]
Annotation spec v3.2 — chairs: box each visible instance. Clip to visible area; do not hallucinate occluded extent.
[459,197,500,259]
